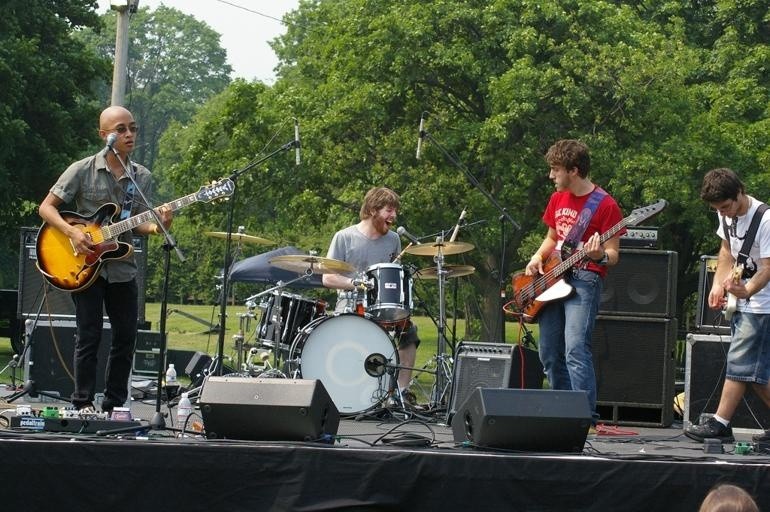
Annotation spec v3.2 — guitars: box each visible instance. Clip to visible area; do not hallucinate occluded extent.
[719,257,754,320]
[36,178,237,292]
[512,200,665,325]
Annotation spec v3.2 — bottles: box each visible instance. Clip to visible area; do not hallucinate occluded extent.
[176,392,193,440]
[165,363,178,388]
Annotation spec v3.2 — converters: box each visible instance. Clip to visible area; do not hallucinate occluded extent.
[16,405,32,417]
[704,439,723,454]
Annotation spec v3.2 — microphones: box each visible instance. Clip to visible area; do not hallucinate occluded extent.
[103,133,117,157]
[416,118,425,160]
[295,121,300,166]
[449,211,467,242]
[397,226,422,245]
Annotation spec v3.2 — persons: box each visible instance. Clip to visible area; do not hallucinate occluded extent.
[524,140,627,435]
[323,187,421,409]
[683,168,770,451]
[698,483,760,511]
[39,106,173,417]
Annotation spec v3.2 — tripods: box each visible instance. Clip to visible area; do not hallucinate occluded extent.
[96,148,206,436]
[7,289,71,403]
[403,252,453,416]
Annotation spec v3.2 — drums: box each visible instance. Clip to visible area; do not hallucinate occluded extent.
[255,290,327,354]
[288,313,399,414]
[364,263,413,325]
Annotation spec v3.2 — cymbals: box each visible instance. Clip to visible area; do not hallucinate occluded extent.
[270,255,355,275]
[412,266,476,280]
[405,241,476,256]
[204,230,277,245]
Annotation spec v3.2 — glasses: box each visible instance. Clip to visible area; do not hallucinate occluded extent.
[104,126,139,133]
[707,201,734,214]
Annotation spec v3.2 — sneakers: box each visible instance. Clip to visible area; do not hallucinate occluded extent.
[683,417,736,442]
[752,429,770,444]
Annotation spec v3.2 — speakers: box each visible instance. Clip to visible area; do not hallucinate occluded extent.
[446,339,545,426]
[598,249,679,318]
[695,255,731,335]
[198,377,341,445]
[23,319,133,409]
[592,315,678,426]
[683,333,770,443]
[16,227,148,325]
[451,387,592,452]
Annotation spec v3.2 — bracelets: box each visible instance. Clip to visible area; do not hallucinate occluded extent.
[595,252,609,265]
[153,225,160,236]
